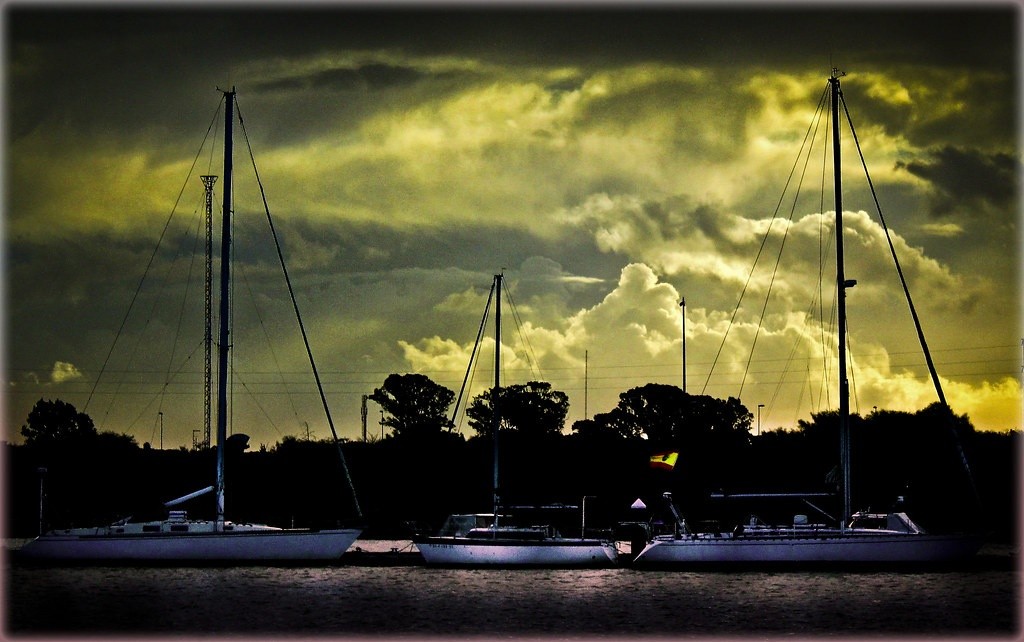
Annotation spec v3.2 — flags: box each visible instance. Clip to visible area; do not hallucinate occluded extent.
[649,450,680,469]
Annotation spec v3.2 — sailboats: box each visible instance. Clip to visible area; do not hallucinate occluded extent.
[6,84,362,563]
[408,272,624,565]
[589,296,717,555]
[642,65,976,570]
